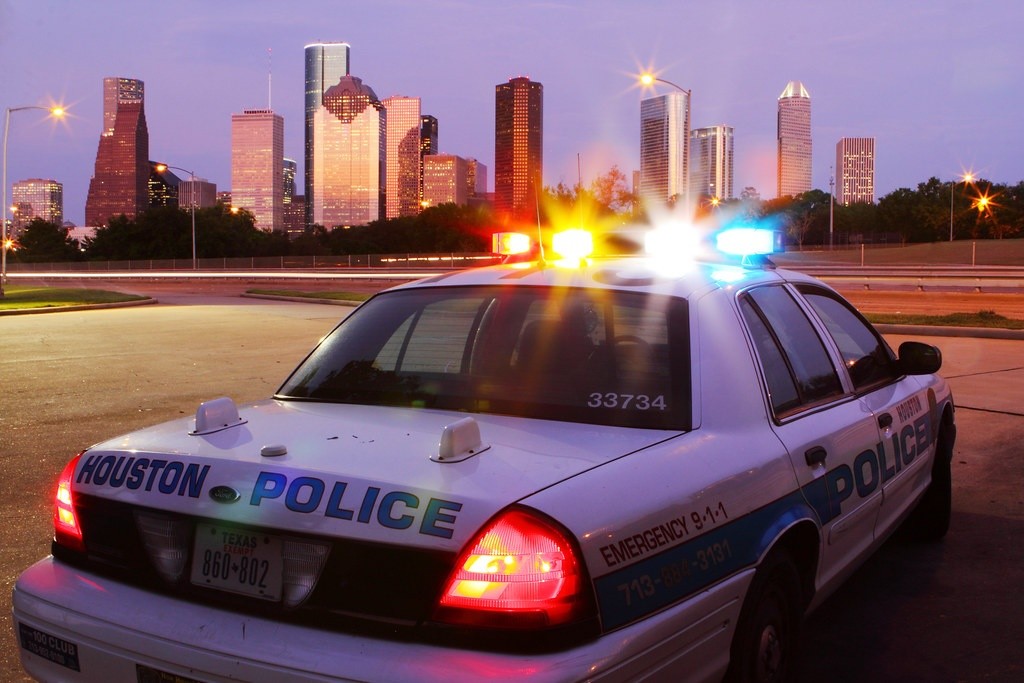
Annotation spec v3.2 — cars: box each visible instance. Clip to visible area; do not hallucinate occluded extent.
[12,231,956,683]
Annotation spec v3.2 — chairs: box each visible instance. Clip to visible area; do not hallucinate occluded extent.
[510,322,609,420]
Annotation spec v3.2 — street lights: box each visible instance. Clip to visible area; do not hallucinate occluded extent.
[829,166,835,251]
[2,106,64,284]
[157,165,198,270]
[642,76,691,217]
[950,175,971,241]
